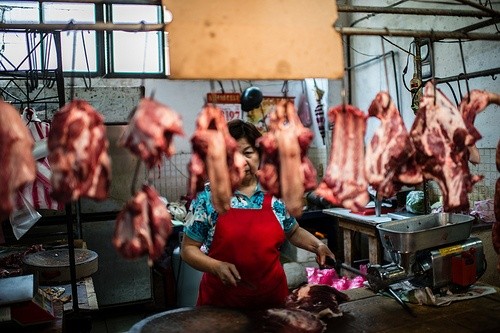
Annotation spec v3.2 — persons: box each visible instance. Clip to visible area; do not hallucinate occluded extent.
[181,119,336,310]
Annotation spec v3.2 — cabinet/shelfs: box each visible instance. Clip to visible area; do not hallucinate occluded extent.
[0,22,168,333]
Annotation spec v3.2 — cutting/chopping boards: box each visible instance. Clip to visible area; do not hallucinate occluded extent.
[141,308,256,333]
[22,248,98,284]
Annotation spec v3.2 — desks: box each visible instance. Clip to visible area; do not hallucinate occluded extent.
[323,208,393,279]
[324,227,500,333]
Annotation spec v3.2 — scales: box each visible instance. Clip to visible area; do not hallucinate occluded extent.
[1,269,55,325]
